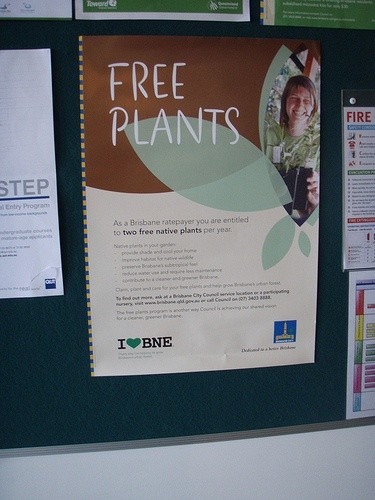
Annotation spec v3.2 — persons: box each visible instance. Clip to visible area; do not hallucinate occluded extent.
[264,74,318,223]
